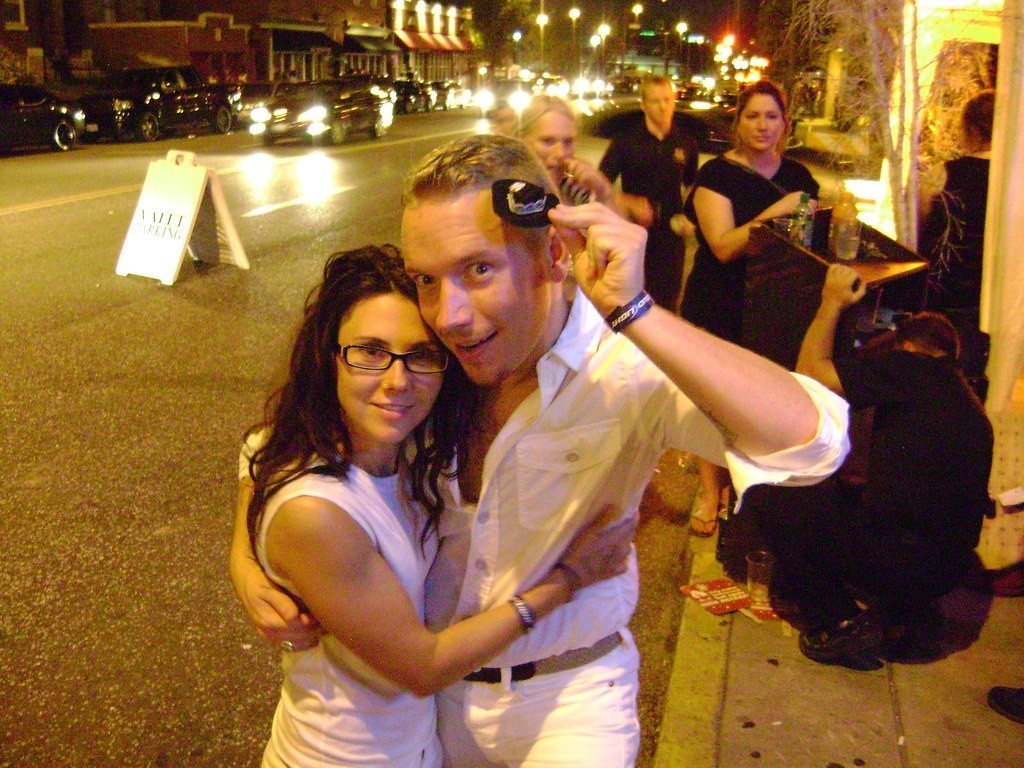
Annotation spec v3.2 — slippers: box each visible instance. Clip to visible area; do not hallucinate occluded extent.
[687,513,717,538]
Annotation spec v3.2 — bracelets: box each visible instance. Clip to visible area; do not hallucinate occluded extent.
[605,290,655,335]
[509,595,534,635]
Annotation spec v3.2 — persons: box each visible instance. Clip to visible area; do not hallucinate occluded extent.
[918,87,995,304]
[248,243,640,768]
[601,75,697,313]
[226,134,852,768]
[758,263,995,661]
[485,94,621,216]
[682,79,832,345]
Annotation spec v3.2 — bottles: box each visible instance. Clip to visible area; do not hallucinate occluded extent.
[827,192,857,252]
[789,192,815,252]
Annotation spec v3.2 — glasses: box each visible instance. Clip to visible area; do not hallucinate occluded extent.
[331,342,451,373]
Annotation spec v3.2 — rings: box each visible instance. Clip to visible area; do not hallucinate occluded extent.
[281,641,292,651]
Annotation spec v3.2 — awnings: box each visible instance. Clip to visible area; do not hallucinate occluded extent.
[344,35,402,53]
[394,29,474,54]
[272,28,343,54]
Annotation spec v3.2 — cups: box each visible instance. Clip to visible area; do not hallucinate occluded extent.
[833,218,862,260]
[773,217,791,235]
[746,550,775,603]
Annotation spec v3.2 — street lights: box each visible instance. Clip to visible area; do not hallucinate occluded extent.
[568,7,587,74]
[536,13,552,66]
[593,24,615,80]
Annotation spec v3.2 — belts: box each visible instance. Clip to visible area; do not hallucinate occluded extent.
[460,630,623,684]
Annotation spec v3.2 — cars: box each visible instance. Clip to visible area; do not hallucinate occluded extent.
[0,82,86,155]
[394,78,476,114]
[488,75,615,104]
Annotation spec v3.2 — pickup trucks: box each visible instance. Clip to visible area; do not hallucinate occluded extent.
[78,63,246,142]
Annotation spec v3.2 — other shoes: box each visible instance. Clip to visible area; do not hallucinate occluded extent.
[988,685,1024,726]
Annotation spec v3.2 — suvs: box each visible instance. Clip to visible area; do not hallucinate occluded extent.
[257,74,397,145]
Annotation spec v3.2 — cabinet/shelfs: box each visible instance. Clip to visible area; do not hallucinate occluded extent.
[716,206,931,583]
